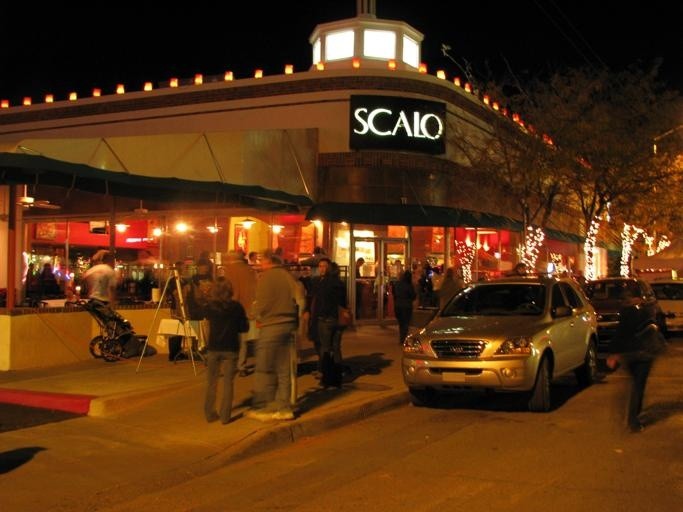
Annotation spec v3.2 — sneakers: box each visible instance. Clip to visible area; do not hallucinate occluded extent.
[243,407,296,422]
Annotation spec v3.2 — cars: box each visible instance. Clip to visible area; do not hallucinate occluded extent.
[402,273,683,412]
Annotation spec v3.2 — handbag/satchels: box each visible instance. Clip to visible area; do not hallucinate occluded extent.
[337,304,355,329]
[634,322,669,358]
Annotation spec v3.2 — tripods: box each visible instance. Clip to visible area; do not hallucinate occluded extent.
[136,264,198,377]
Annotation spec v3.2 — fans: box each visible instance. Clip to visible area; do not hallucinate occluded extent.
[13,185,62,211]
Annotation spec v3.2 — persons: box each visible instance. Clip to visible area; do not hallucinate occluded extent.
[185,265,209,354]
[616,278,669,433]
[310,262,348,389]
[16,248,160,303]
[438,268,458,310]
[303,259,328,379]
[246,249,305,411]
[355,257,371,320]
[221,250,254,378]
[507,263,527,277]
[196,250,215,281]
[167,261,186,361]
[392,269,417,344]
[238,246,330,275]
[199,277,248,423]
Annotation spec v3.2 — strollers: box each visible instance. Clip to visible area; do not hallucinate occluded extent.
[79,299,147,362]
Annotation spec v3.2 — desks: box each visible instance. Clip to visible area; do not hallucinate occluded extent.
[158,318,210,366]
